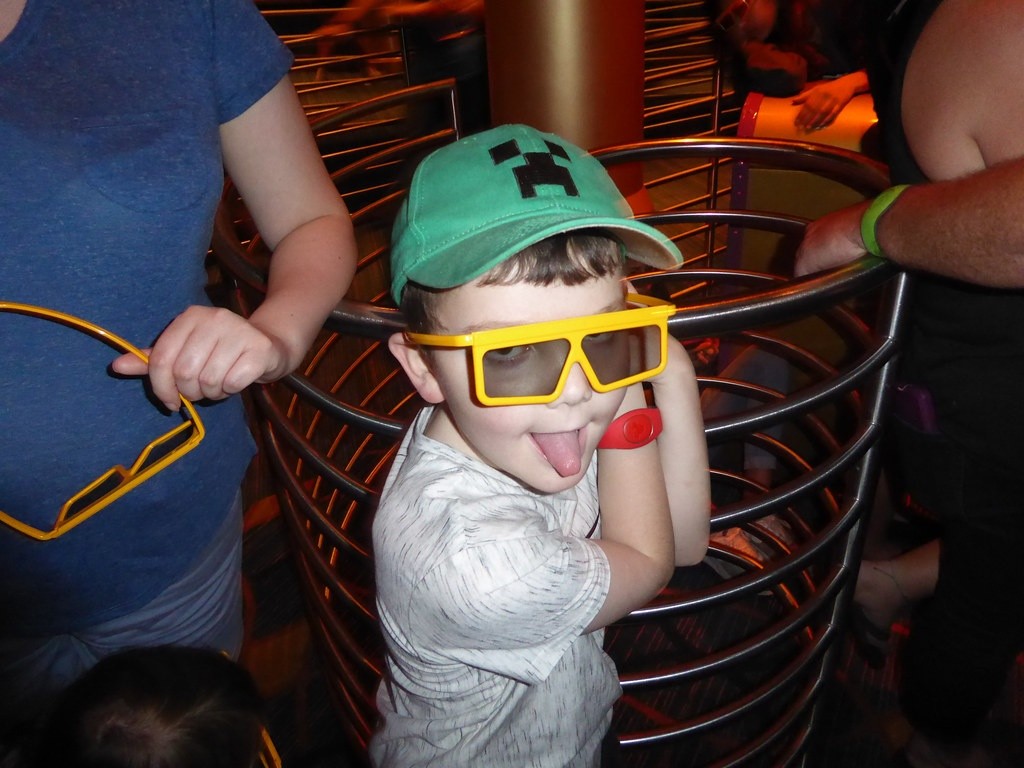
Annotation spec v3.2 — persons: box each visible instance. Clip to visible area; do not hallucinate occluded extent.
[18,646,282,768]
[366,125,711,768]
[0,0,359,768]
[698,0,1024,768]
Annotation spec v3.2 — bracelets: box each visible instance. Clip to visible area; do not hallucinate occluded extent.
[595,408,663,449]
[861,185,911,257]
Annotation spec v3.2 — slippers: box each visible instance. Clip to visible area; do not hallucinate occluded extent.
[848,600,889,670]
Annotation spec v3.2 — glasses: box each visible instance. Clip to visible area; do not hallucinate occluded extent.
[0,300,205,540]
[405,292,676,406]
[256,724,281,768]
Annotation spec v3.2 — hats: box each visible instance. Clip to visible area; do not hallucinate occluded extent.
[390,124,683,306]
